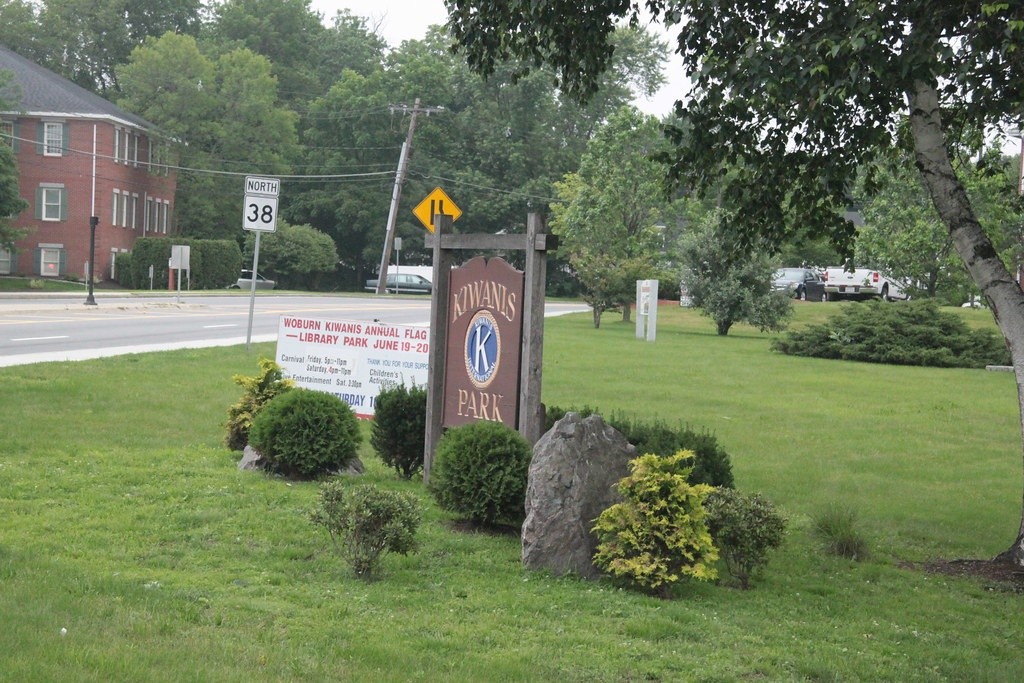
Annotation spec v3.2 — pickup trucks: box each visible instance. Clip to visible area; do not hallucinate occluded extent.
[823,256,909,302]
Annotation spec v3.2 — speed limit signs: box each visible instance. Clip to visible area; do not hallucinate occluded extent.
[243,177,281,232]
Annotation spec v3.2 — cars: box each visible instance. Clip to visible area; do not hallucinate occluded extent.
[364,273,432,294]
[228,269,277,290]
[769,267,827,302]
